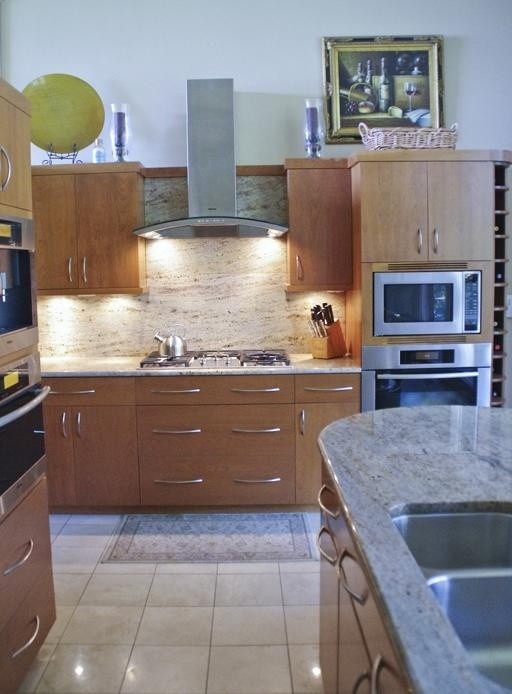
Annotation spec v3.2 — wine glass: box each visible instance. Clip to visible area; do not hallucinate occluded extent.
[404,81,416,115]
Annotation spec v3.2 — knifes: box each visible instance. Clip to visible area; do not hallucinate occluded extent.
[308,302,334,335]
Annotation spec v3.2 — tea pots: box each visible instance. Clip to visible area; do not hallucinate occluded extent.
[152,324,188,356]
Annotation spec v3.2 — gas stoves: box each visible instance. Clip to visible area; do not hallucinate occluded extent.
[138,347,292,370]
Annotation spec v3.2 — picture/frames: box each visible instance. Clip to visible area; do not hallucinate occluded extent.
[322,34,447,146]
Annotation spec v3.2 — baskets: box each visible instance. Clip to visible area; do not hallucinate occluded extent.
[358,121,459,150]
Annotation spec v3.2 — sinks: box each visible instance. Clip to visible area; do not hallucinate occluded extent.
[426,576,512,686]
[392,513,512,568]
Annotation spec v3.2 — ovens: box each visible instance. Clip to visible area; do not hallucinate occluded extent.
[360,343,491,410]
[0,210,57,518]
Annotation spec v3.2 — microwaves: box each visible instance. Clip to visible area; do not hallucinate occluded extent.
[371,269,480,335]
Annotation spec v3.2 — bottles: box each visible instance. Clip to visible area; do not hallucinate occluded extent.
[356,60,364,83]
[378,56,393,113]
[364,58,375,94]
[339,87,377,106]
[92,137,106,162]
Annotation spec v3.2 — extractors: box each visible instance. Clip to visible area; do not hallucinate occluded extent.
[132,76,289,243]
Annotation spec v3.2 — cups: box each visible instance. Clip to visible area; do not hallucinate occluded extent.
[110,102,130,163]
[302,97,325,158]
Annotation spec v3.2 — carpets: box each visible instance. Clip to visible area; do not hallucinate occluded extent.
[101,510,316,565]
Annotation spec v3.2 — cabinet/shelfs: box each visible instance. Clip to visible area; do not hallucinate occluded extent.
[295,374,361,513]
[352,150,494,265]
[40,375,139,515]
[319,459,410,692]
[31,162,150,296]
[283,158,353,294]
[137,375,296,514]
[0,476,57,693]
[0,78,33,218]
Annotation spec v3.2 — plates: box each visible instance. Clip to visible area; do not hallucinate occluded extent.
[22,72,105,153]
